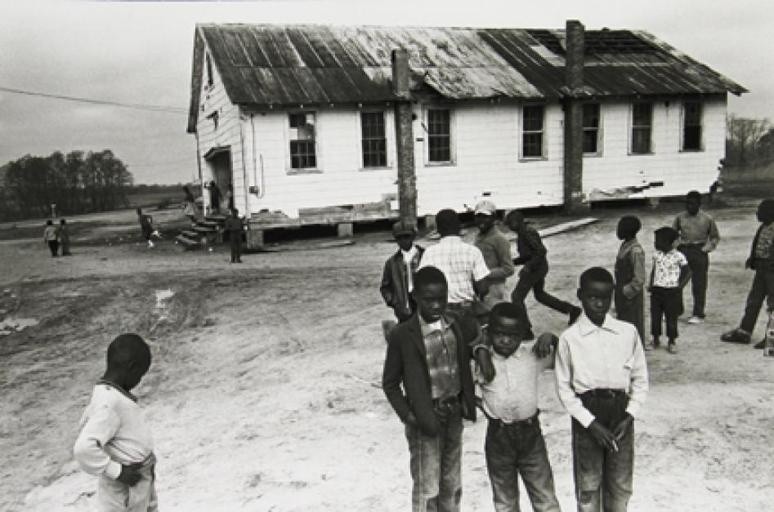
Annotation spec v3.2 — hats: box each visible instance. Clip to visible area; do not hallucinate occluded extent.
[475,200,495,216]
[392,221,415,236]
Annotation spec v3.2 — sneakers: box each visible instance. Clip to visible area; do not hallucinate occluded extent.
[689,317,705,323]
[646,341,659,350]
[667,343,677,353]
[720,328,750,342]
[568,308,582,326]
[754,340,765,348]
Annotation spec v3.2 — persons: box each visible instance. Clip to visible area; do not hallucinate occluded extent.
[554,267,650,510]
[136,207,162,249]
[614,214,646,349]
[225,208,243,263]
[381,201,582,340]
[44,220,59,256]
[382,265,494,511]
[221,182,232,214]
[203,179,223,216]
[470,302,560,511]
[72,332,159,512]
[720,198,774,349]
[182,185,200,227]
[379,199,650,512]
[669,189,721,323]
[58,219,72,255]
[647,225,694,354]
